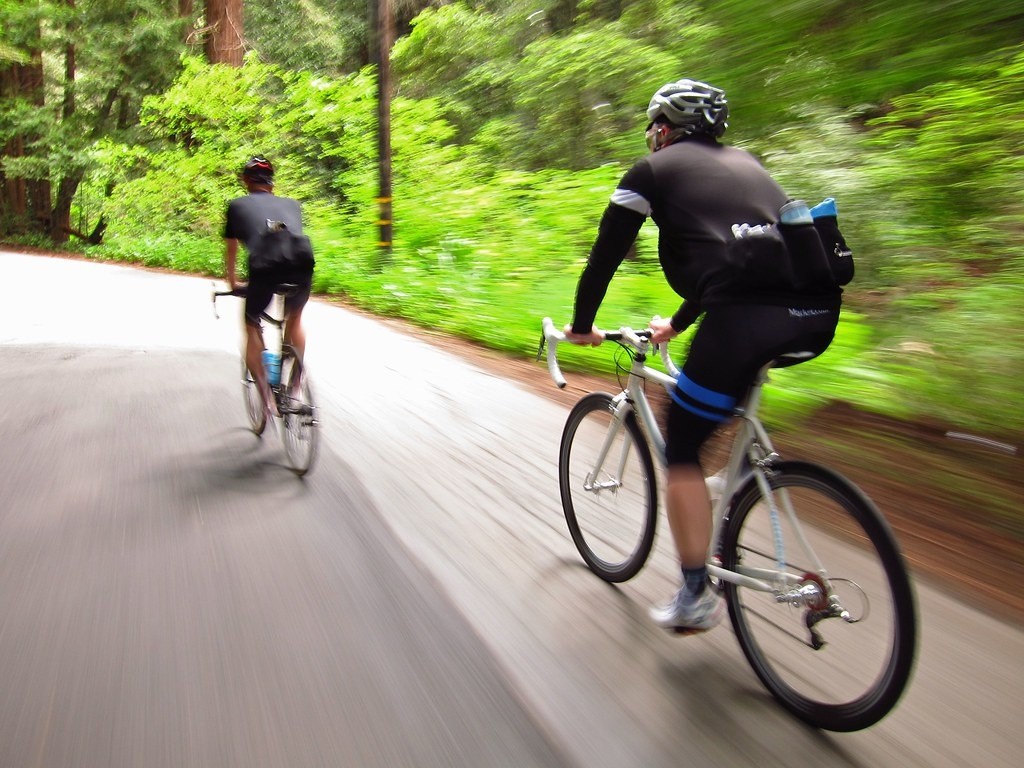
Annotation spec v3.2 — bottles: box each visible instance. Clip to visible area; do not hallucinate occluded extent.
[779,199,813,223]
[260,348,281,384]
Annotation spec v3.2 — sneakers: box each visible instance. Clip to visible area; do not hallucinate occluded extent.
[704,466,727,501]
[648,585,726,632]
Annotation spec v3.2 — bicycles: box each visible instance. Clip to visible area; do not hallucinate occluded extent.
[534,320,918,735]
[211,288,325,475]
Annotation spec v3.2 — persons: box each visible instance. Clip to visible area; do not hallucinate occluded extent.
[562,76,857,632]
[218,154,317,420]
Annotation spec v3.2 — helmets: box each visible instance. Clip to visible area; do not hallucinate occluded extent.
[646,78,729,138]
[244,158,273,183]
